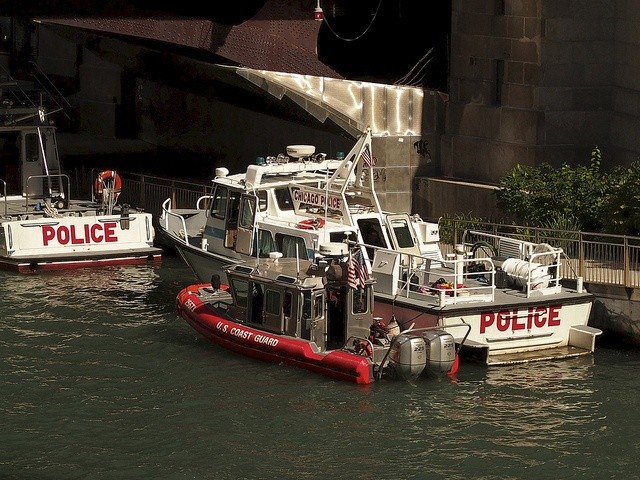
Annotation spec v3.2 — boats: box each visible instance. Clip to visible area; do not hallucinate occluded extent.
[0,93,165,276]
[159,128,604,366]
[177,256,471,384]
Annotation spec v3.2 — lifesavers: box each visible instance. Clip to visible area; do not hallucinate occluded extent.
[355,342,373,360]
[94,170,122,203]
[297,218,325,229]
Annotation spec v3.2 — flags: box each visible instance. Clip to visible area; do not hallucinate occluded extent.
[347,265,358,290]
[352,249,369,288]
